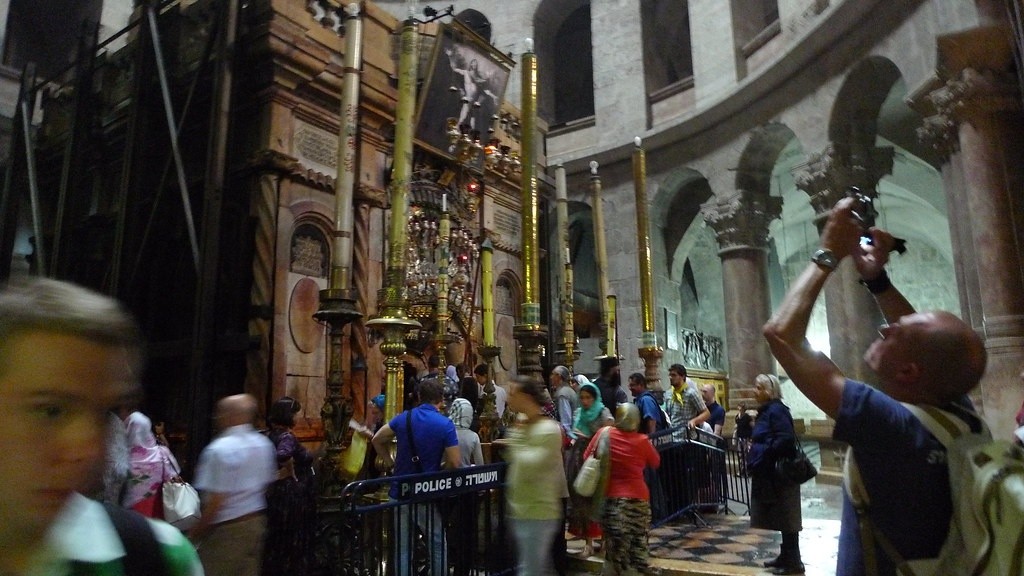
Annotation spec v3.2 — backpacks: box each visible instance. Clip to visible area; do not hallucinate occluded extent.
[843,395,1023,576]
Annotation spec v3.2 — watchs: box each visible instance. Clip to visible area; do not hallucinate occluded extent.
[811,248,837,271]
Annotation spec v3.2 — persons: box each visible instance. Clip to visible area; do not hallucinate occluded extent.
[763,197,993,576]
[121,365,725,576]
[733,404,754,476]
[750,374,806,575]
[0,278,205,576]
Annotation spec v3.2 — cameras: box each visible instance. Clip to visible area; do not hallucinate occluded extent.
[843,185,875,248]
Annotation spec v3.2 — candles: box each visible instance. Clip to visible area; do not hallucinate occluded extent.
[442,194,446,212]
[566,248,570,264]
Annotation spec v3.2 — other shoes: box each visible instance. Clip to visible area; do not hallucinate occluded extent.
[580,545,593,559]
[771,559,805,575]
[764,553,785,567]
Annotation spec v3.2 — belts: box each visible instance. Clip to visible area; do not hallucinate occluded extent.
[213,508,268,528]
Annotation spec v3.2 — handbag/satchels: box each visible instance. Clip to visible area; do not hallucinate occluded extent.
[158,446,201,529]
[574,425,611,497]
[774,445,819,485]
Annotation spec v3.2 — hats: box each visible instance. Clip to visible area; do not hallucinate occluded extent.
[428,355,439,368]
[600,357,621,369]
[371,395,385,413]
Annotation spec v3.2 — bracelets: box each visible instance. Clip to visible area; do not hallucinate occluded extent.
[862,268,890,294]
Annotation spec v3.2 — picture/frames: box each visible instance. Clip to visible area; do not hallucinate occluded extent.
[664,308,679,351]
[412,22,510,176]
